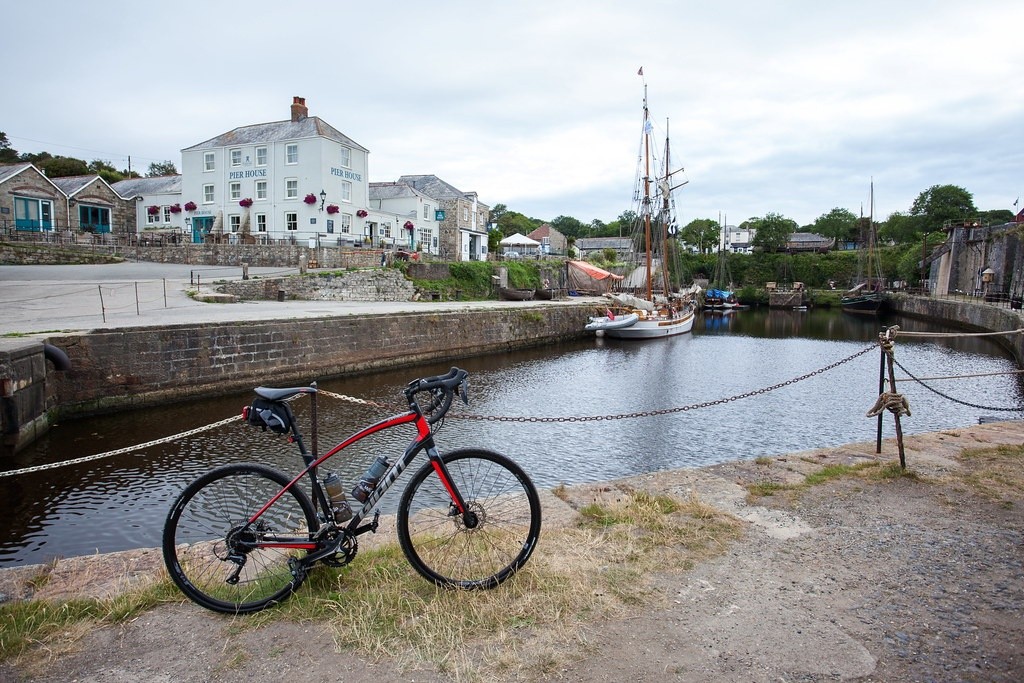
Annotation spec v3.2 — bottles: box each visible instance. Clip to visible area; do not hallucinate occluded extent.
[350,455,389,504]
[322,472,353,524]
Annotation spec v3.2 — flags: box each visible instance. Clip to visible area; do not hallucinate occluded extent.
[435,210,445,221]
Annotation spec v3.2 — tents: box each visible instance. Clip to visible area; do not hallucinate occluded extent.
[566,259,624,296]
[498,232,541,255]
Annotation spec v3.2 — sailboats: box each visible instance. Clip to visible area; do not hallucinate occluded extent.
[583,65,702,339]
[840,176,887,313]
[701,212,741,309]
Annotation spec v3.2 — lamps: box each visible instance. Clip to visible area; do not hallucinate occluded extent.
[320,189,327,204]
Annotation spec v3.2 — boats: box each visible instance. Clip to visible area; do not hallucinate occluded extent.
[536,287,570,301]
[499,286,537,301]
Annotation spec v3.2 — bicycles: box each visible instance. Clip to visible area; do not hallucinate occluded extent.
[158,364,543,617]
